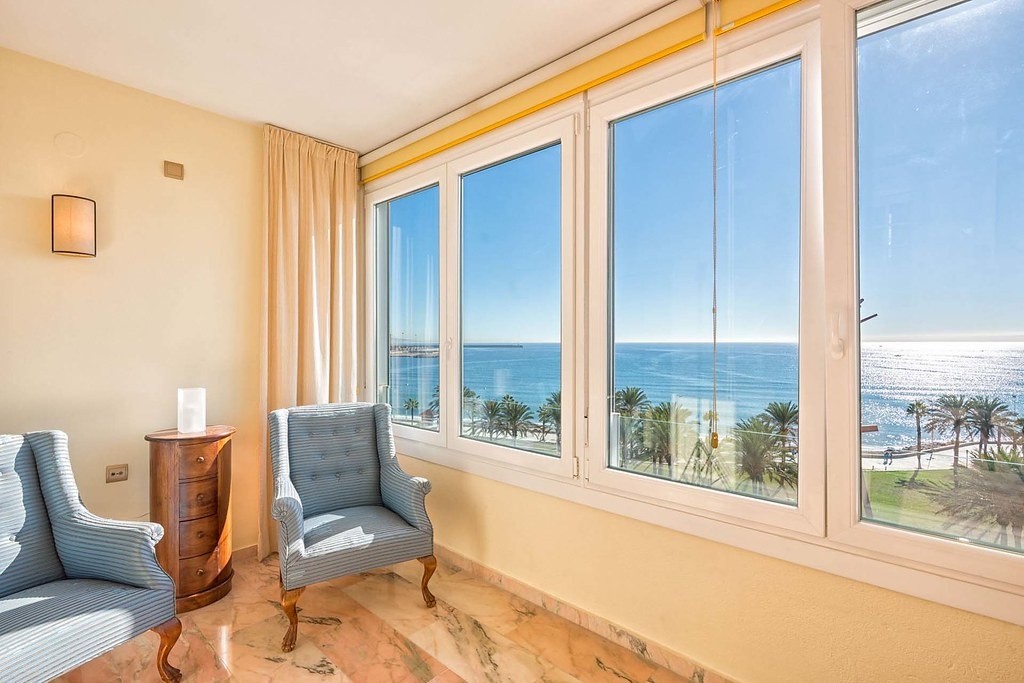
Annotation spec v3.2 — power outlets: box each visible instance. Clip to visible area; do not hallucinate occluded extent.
[106,463,128,483]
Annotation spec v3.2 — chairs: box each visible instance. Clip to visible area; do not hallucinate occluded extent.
[268,402,437,651]
[0,428,183,683]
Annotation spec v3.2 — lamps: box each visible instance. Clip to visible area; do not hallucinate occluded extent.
[51,194,97,258]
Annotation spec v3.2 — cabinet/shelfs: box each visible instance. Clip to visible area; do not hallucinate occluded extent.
[144,424,237,614]
